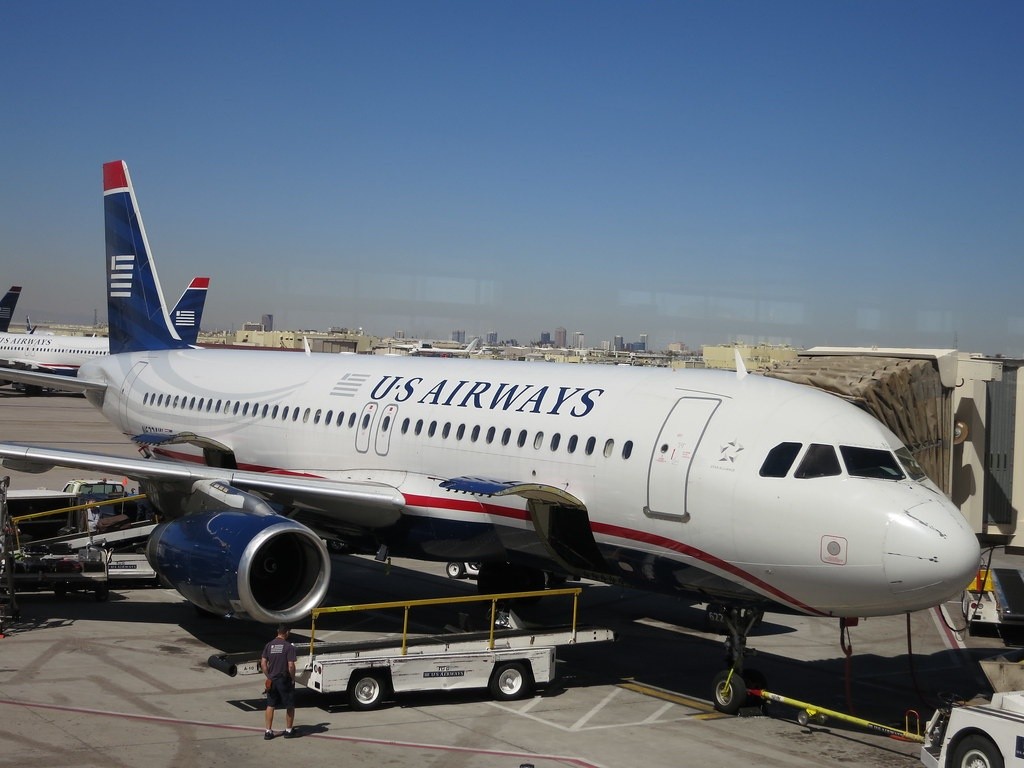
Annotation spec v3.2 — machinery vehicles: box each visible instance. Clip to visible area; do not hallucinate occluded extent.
[62,479,140,522]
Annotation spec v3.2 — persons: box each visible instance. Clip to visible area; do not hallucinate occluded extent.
[131,488,135,494]
[86,500,101,529]
[261,623,299,740]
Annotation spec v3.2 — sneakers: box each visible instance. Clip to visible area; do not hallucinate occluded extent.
[264,732,274,740]
[284,728,303,738]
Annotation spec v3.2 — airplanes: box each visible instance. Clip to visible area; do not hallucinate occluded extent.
[0,160,985,714]
